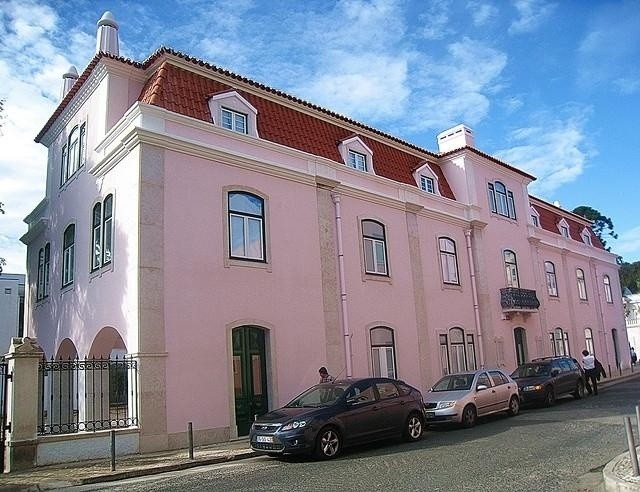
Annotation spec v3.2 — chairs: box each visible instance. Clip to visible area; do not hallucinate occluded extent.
[334,385,344,401]
[454,378,466,386]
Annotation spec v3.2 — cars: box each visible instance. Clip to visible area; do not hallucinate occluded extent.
[423,372,520,424]
[247,378,428,455]
[508,356,587,404]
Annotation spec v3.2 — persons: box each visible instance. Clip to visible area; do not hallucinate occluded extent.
[319,367,336,403]
[582,350,598,396]
